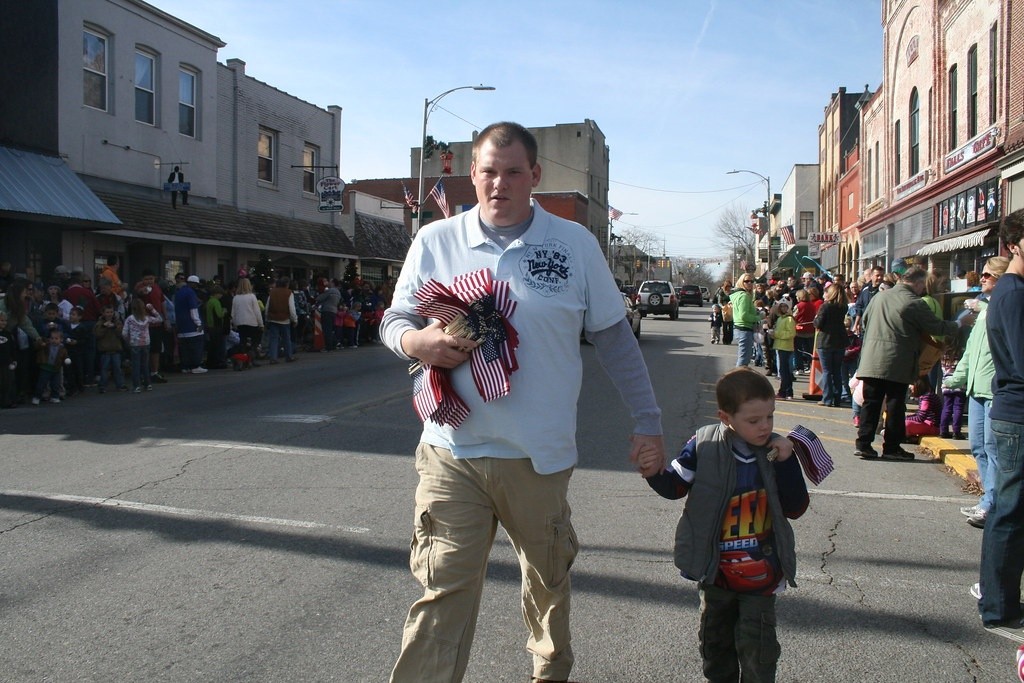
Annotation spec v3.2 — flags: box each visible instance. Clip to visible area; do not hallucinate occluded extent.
[430,177,451,219]
[780,225,796,245]
[767,424,836,485]
[608,204,623,221]
[408,267,519,430]
[746,227,765,235]
[401,181,418,214]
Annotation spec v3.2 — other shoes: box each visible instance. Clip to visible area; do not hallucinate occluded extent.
[796,367,811,376]
[0,333,380,417]
[907,436,920,443]
[971,582,982,599]
[853,416,860,427]
[765,368,773,376]
[711,339,732,345]
[775,395,793,401]
[984,617,1024,643]
[940,431,966,440]
[818,401,840,407]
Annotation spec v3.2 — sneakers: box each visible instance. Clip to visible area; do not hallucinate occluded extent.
[966,508,987,528]
[883,447,915,460]
[961,504,980,516]
[854,446,878,458]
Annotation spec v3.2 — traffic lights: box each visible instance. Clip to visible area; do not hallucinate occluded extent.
[636,259,640,267]
[667,261,670,268]
[662,260,665,269]
[657,260,660,267]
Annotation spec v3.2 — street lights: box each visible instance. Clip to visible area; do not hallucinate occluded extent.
[605,208,638,268]
[418,84,495,230]
[612,236,623,279]
[726,169,773,271]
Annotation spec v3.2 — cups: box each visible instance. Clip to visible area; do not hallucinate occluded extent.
[768,329,774,334]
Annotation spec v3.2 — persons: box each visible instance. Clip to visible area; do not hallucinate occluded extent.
[169,166,190,209]
[940,255,1011,528]
[377,123,667,683]
[635,369,810,683]
[853,263,978,459]
[979,208,1024,645]
[711,212,989,439]
[0,256,396,408]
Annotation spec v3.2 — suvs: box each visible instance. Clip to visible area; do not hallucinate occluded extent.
[636,281,679,320]
[619,280,710,320]
[679,285,704,307]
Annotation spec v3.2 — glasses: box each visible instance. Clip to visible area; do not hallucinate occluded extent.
[981,273,991,279]
[181,277,185,280]
[744,280,753,283]
[28,288,33,291]
[83,279,91,283]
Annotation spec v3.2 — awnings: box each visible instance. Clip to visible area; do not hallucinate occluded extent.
[915,229,992,258]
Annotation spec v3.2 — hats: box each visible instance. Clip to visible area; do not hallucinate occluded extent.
[56,265,71,274]
[187,275,200,283]
[892,259,907,273]
[800,272,813,278]
[47,280,62,293]
[825,281,832,288]
[817,273,828,279]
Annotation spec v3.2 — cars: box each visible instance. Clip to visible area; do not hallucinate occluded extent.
[619,292,642,339]
